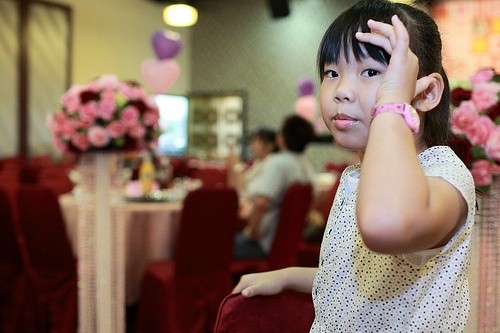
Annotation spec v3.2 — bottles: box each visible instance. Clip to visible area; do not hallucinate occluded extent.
[139,153,156,202]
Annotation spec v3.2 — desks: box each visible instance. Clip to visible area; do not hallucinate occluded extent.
[59,193,252,276]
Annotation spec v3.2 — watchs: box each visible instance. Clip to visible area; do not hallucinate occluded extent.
[371,102,421,134]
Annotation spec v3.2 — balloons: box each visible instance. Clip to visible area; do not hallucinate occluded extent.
[296,95,316,120]
[299,78,314,96]
[152,29,182,60]
[143,59,181,93]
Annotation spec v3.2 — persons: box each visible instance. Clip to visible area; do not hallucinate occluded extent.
[232,115,316,259]
[228,130,278,193]
[228,0,475,332]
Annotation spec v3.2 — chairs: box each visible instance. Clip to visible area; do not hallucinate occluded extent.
[0,157,348,333]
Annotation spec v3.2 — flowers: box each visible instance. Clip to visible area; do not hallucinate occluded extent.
[47,76,159,163]
[449,69,500,193]
